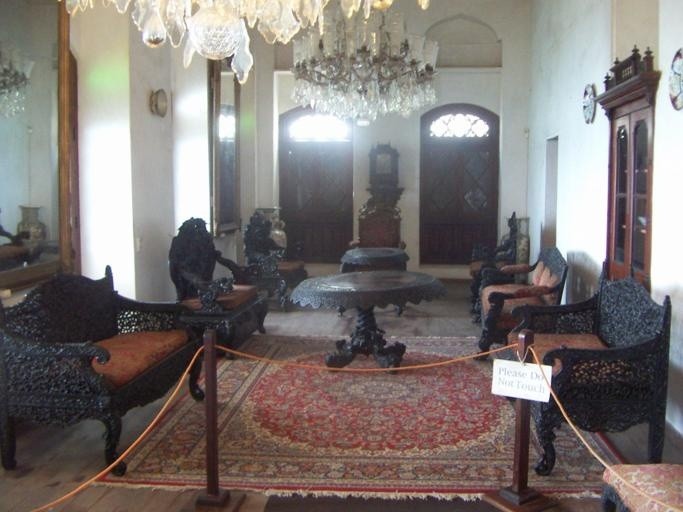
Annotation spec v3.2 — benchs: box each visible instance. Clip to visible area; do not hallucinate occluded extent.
[508,262,671,474]
[471,246,567,358]
[470,211,530,310]
[243,210,306,308]
[4,267,207,476]
[169,217,268,358]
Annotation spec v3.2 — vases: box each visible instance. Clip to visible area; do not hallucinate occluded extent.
[16,205,47,263]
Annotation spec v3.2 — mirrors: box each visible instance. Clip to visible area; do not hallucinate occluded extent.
[0,0,72,294]
[207,59,242,238]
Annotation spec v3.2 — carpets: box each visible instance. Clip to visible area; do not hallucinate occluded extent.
[90,333,631,500]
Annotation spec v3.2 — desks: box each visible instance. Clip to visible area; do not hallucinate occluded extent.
[288,246,446,375]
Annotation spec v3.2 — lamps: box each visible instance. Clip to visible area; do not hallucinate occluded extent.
[289,5,437,129]
[65,1,397,85]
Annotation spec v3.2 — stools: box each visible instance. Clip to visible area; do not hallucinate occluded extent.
[602,461,683,512]
